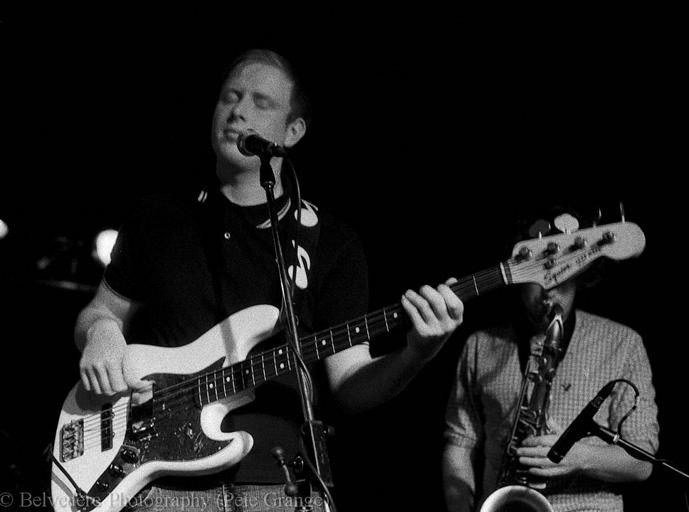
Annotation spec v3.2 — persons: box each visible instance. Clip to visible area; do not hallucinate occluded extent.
[442,208,662,512]
[71,47,465,511]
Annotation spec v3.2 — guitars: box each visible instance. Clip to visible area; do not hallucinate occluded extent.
[51,203,645,511]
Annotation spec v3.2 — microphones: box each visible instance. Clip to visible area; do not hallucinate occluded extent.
[236,127,288,158]
[548,381,617,465]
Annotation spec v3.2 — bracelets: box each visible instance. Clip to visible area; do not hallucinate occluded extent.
[85,312,121,334]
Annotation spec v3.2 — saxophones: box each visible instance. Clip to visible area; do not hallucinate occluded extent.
[480,297,565,511]
